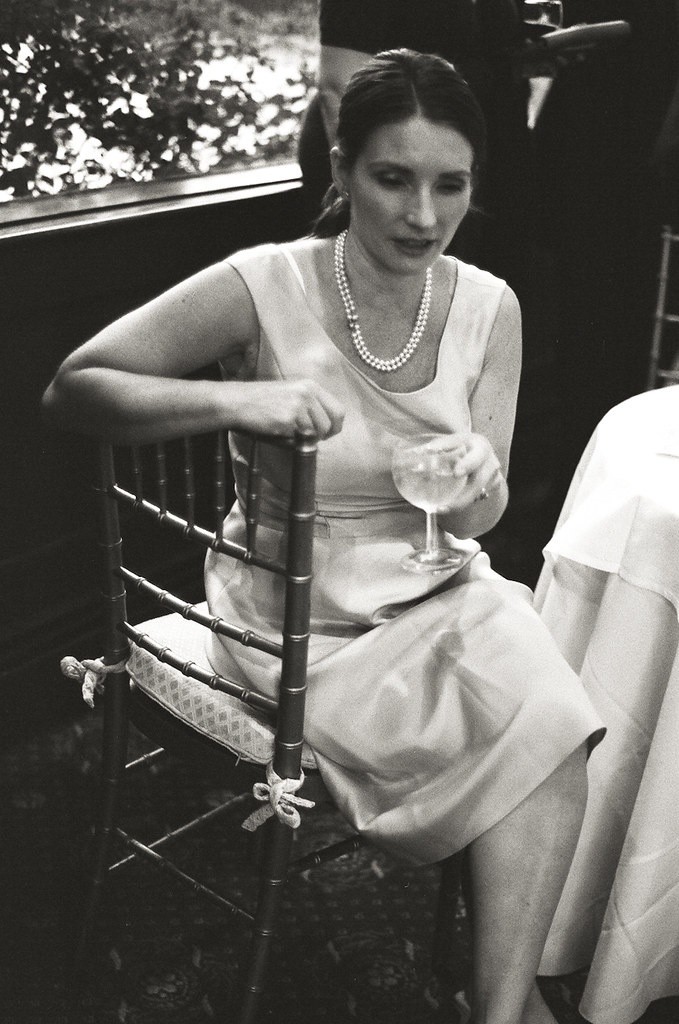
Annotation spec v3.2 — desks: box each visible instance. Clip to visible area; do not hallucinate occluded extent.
[534,384,679,1024]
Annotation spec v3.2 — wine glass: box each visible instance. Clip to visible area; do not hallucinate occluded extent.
[390,433,469,575]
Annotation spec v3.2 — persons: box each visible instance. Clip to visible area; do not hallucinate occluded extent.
[43,48,612,1023]
[297,0,679,514]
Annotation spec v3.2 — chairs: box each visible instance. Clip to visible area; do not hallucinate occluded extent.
[83,420,460,1024]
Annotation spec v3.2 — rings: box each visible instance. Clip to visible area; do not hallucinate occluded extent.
[478,488,488,499]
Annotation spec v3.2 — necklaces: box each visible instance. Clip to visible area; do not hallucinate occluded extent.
[333,229,432,372]
